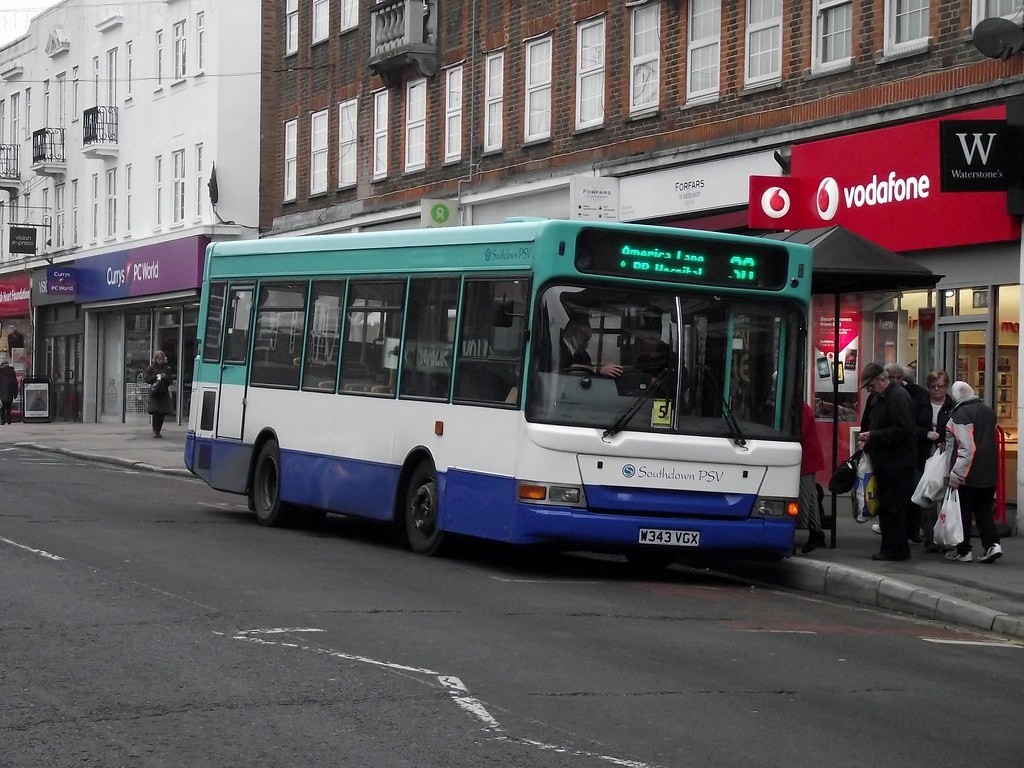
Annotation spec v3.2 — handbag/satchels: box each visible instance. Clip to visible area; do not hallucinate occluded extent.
[910,445,951,507]
[933,486,964,547]
[851,451,879,523]
[828,449,863,495]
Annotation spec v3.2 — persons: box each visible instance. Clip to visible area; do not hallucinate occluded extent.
[30,390,45,410]
[858,362,1004,565]
[144,350,172,439]
[542,308,689,416]
[769,393,826,554]
[0,360,18,424]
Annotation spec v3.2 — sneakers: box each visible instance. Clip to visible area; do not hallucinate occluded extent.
[946,549,972,562]
[978,543,1002,563]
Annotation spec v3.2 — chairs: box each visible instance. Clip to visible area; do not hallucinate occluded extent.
[218,325,454,398]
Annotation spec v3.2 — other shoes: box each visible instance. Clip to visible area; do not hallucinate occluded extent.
[872,552,911,561]
[907,530,923,543]
[801,529,825,554]
[871,524,882,533]
[153,430,162,438]
[923,542,942,553]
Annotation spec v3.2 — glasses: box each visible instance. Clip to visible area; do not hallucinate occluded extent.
[929,383,944,389]
[864,383,875,390]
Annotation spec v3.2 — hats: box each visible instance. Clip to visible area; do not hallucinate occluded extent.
[860,363,884,389]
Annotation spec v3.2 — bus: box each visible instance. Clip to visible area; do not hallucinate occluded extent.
[179,216,813,558]
